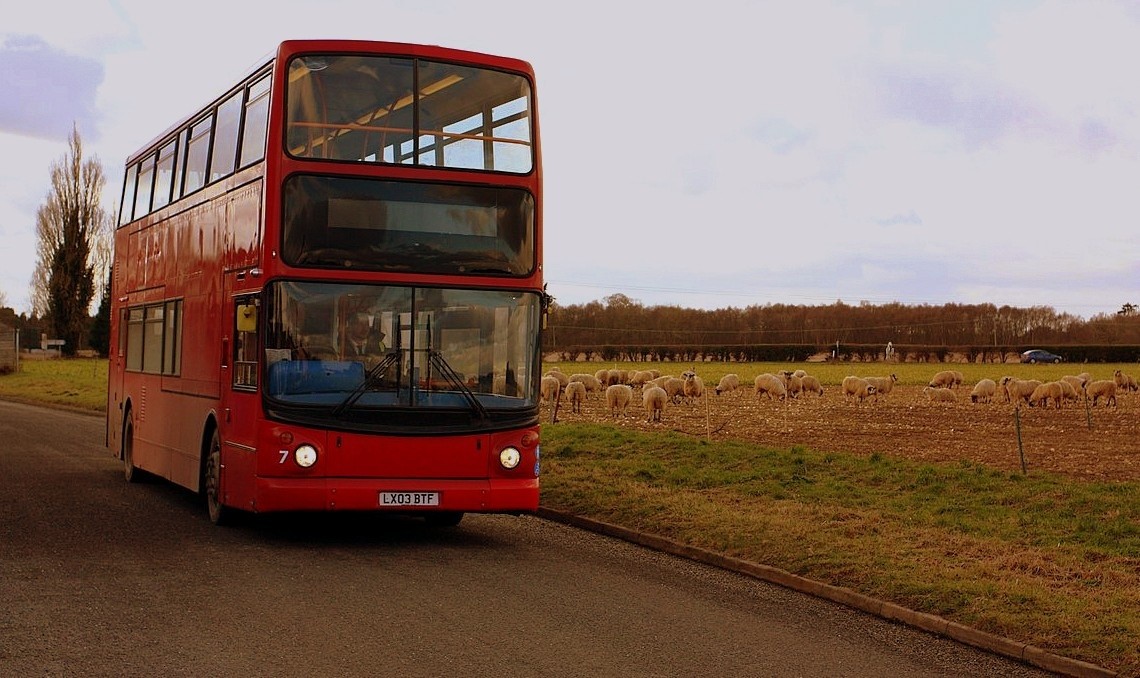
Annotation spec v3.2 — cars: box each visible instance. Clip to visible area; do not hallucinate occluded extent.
[1021,349,1062,365]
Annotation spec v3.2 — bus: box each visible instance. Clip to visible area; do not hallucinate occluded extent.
[104,38,554,526]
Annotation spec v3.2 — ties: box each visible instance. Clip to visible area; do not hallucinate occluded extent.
[357,344,363,356]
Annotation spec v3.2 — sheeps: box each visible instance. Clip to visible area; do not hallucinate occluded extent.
[1028,382,1063,409]
[923,386,957,406]
[606,384,633,418]
[1113,370,1139,395]
[642,381,667,423]
[1056,373,1090,408]
[970,379,996,404]
[754,370,824,402]
[1082,378,1117,407]
[862,373,898,403]
[951,371,963,390]
[465,367,704,414]
[714,374,739,396]
[842,375,876,409]
[999,375,1043,411]
[929,371,956,389]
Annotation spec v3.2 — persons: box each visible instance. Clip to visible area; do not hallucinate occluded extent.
[344,312,386,363]
